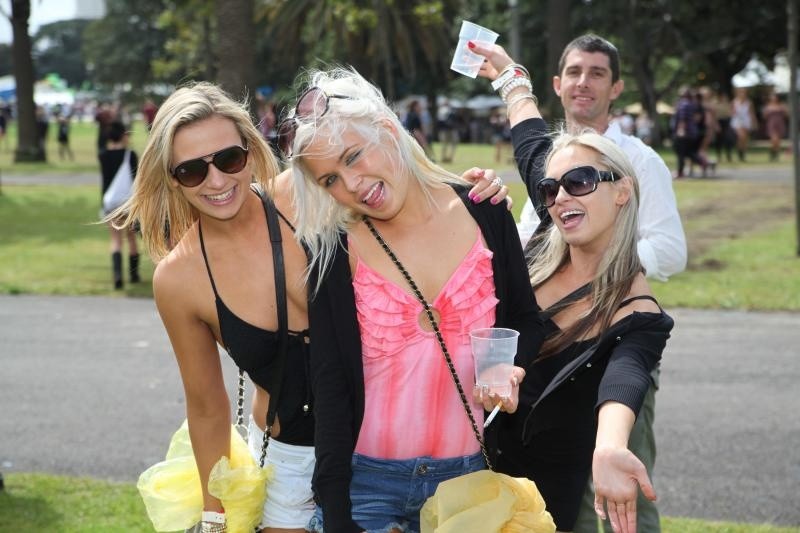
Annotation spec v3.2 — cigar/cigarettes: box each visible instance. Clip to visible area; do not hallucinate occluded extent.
[483,400,503,427]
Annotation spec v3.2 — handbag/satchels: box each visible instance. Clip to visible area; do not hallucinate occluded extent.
[102,148,136,217]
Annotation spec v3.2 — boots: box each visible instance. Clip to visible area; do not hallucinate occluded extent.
[113,252,122,289]
[128,253,141,282]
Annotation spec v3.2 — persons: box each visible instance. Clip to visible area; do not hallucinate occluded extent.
[0,97,284,290]
[517,34,688,533]
[86,82,512,533]
[396,83,790,178]
[275,64,545,533]
[465,39,675,533]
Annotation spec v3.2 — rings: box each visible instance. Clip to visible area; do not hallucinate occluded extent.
[492,178,503,188]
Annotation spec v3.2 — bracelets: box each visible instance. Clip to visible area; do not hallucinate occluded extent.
[201,509,227,533]
[491,62,538,118]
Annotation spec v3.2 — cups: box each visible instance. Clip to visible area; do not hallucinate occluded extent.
[470,328,520,399]
[450,19,500,79]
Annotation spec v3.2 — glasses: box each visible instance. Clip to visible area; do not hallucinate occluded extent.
[537,166,621,208]
[169,139,248,188]
[276,86,359,159]
